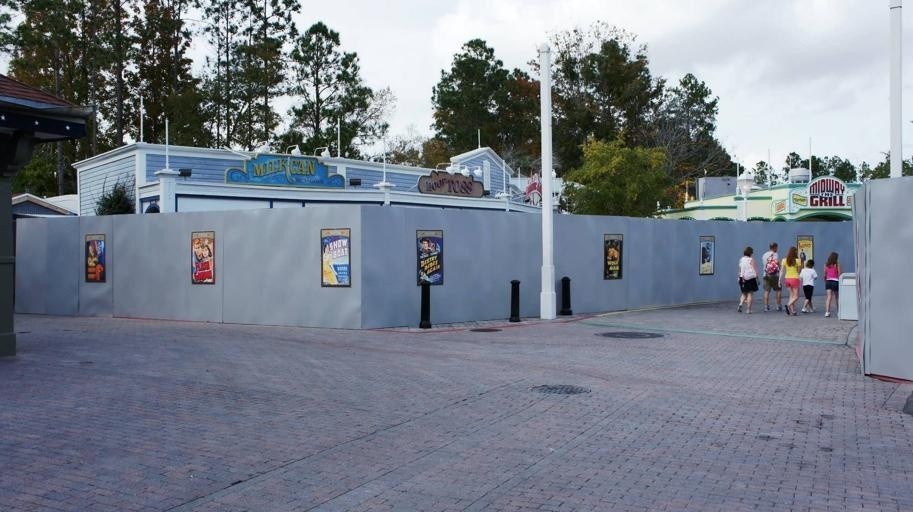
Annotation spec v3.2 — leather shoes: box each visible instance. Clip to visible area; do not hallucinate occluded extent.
[785,305,798,314]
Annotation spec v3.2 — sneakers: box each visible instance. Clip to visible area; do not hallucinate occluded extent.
[775,304,782,311]
[737,303,743,312]
[825,311,831,317]
[801,306,816,312]
[746,309,753,313]
[764,305,772,312]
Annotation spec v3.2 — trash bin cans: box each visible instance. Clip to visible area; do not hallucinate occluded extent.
[838,272,859,321]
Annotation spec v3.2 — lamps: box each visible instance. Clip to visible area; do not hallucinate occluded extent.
[254,141,331,161]
[436,163,482,177]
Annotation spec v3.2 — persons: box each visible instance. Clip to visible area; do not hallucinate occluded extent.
[194,243,213,280]
[799,249,806,269]
[799,260,818,314]
[192,237,204,274]
[322,242,338,284]
[736,246,760,314]
[761,242,783,312]
[605,239,621,277]
[418,237,433,256]
[822,251,841,317]
[702,249,711,263]
[777,246,803,315]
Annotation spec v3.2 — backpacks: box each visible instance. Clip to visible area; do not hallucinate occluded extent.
[764,252,780,275]
